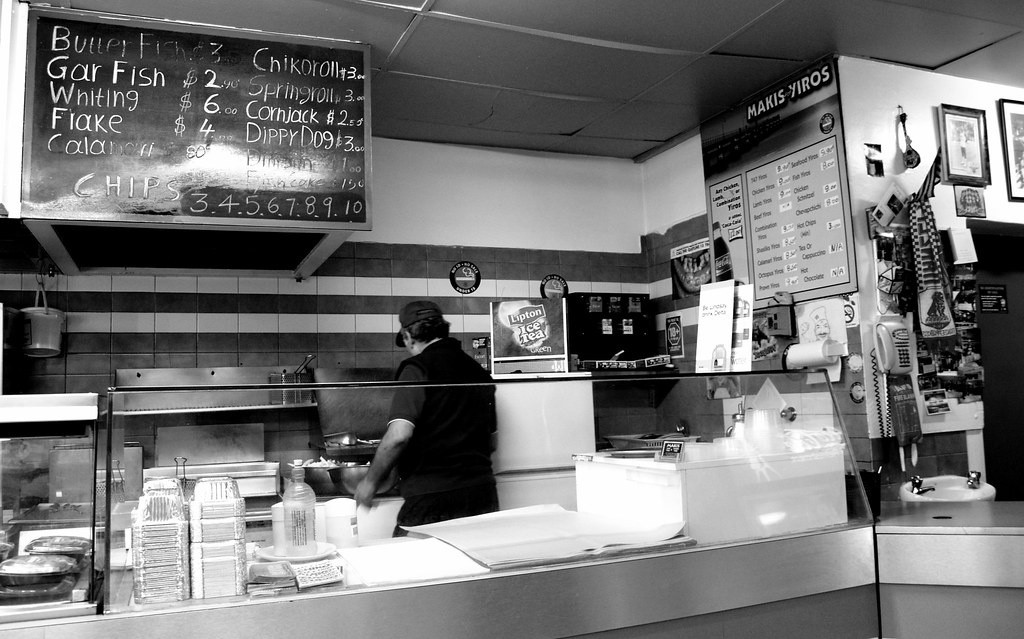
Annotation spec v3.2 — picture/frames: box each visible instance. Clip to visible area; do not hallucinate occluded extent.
[999,98,1024,202]
[936,103,992,189]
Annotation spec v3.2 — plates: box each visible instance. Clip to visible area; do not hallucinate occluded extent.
[257,544,334,561]
[610,450,659,458]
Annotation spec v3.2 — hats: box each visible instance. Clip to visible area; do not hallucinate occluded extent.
[395,301,443,348]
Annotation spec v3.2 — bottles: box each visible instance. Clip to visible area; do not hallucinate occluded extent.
[323,498,358,549]
[282,460,317,555]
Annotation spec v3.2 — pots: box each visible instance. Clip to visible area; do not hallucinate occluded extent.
[301,462,356,494]
[327,464,401,495]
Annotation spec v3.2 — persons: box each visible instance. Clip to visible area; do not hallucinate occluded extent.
[355,301,498,538]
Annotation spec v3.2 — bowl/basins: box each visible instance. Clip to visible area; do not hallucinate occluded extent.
[324,431,357,445]
[743,409,783,454]
[0,523,92,601]
[130,477,245,605]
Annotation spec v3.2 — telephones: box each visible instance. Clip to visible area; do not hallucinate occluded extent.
[872,321,911,375]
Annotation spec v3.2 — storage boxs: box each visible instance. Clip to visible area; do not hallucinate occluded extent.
[572,442,849,548]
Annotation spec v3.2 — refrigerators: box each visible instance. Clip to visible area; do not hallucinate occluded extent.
[494,380,594,511]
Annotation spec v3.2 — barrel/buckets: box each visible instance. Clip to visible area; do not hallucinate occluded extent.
[21,283,65,357]
